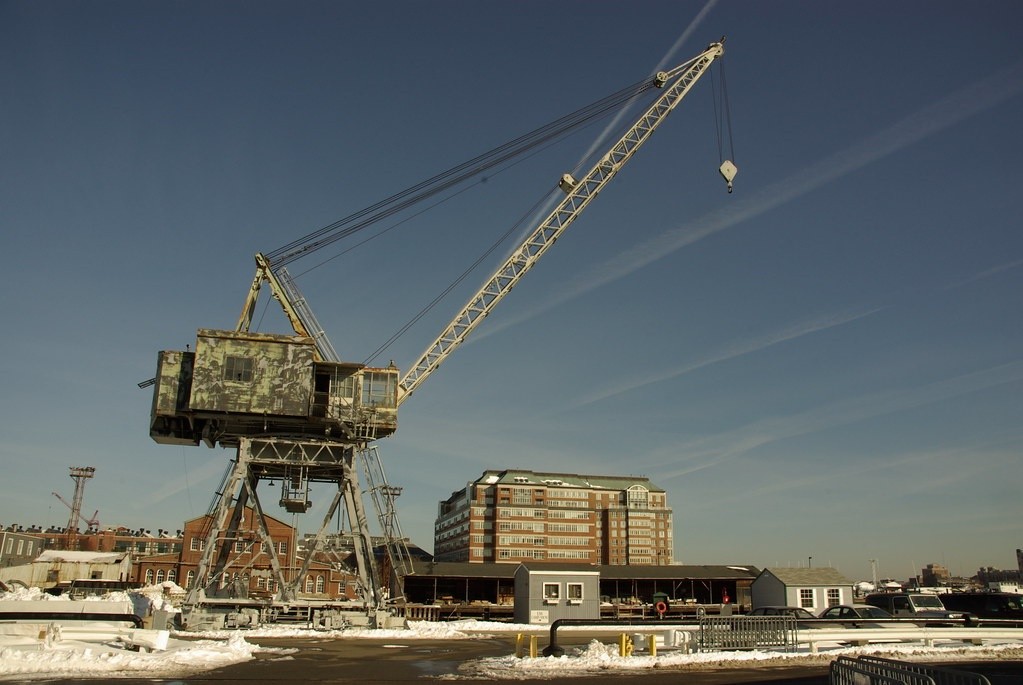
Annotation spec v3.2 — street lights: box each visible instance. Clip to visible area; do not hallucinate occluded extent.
[809,557,813,568]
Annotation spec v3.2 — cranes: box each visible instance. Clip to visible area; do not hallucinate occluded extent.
[147,36,740,624]
[51,490,101,533]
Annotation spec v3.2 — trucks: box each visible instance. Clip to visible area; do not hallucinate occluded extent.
[864,592,946,617]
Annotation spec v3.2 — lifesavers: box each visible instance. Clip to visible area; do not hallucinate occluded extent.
[655,601,667,613]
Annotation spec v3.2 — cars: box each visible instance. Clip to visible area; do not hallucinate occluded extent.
[738,605,846,633]
[818,605,919,627]
